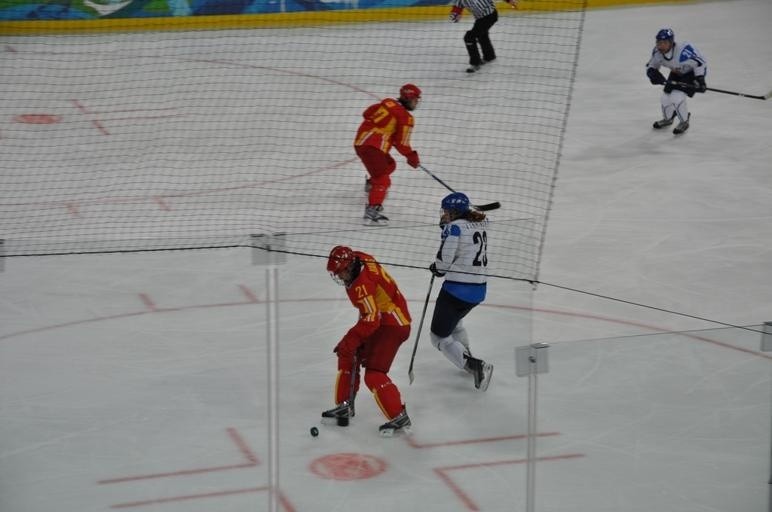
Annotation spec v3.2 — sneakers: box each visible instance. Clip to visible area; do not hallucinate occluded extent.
[380,410,411,430]
[322,403,355,417]
[467,65,482,72]
[463,351,486,388]
[363,207,389,222]
[480,55,495,64]
[654,119,672,128]
[673,122,689,134]
[365,180,373,192]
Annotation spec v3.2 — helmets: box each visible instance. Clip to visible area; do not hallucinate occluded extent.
[401,85,421,102]
[327,246,360,286]
[656,28,673,52]
[440,193,468,220]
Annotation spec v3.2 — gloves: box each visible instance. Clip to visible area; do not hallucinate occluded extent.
[651,75,665,84]
[695,76,706,92]
[334,337,362,359]
[448,15,457,23]
[430,263,445,277]
[407,152,418,168]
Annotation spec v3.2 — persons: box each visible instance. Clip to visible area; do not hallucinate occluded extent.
[645,26,707,135]
[446,0,519,73]
[428,192,488,389]
[353,82,421,222]
[322,245,412,430]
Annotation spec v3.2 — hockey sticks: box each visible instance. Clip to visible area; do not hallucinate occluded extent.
[407,272,434,387]
[663,79,772,102]
[418,163,501,212]
